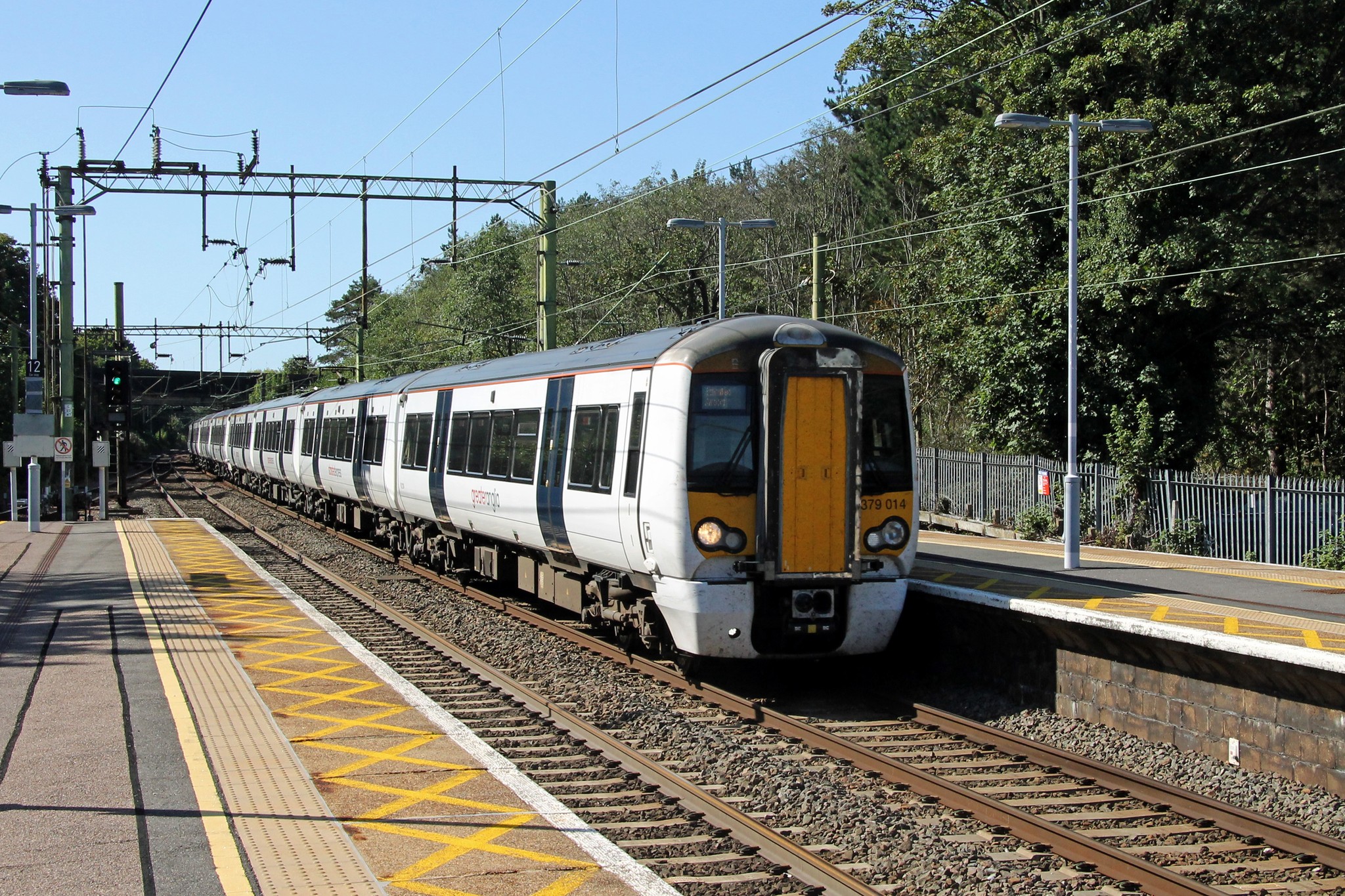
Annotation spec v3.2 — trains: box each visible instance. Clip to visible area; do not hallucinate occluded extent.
[187,313,921,671]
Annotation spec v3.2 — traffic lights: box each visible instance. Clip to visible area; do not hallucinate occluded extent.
[105,359,129,407]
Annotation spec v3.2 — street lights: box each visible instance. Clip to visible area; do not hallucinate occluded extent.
[993,112,1154,581]
[666,217,777,318]
[1,202,97,535]
